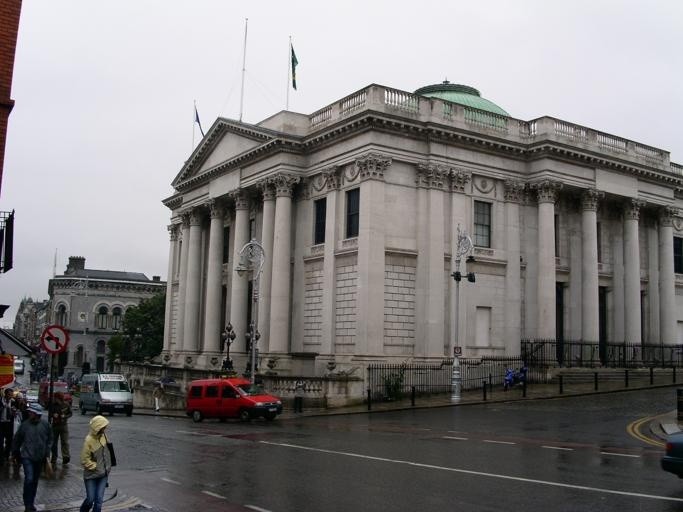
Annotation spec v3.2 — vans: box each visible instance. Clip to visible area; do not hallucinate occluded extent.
[185,377,283,422]
[79,373,133,416]
[38,382,72,408]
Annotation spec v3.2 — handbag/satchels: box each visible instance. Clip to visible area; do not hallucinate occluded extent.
[106,443,117,467]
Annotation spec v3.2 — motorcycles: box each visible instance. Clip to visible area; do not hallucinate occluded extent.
[503,364,527,393]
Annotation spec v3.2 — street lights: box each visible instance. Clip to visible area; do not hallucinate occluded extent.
[221,321,236,371]
[245,319,260,372]
[451,224,475,402]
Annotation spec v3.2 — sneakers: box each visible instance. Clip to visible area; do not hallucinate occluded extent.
[51,456,70,464]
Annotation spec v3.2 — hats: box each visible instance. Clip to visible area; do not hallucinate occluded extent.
[26,403,43,415]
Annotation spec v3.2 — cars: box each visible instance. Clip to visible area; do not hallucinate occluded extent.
[661,430,682,480]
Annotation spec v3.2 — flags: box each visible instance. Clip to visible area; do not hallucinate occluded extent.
[291,43,298,91]
[195,109,204,138]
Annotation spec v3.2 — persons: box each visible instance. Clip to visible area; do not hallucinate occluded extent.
[80,415,113,512]
[293,376,306,413]
[153,383,164,411]
[0,388,73,512]
